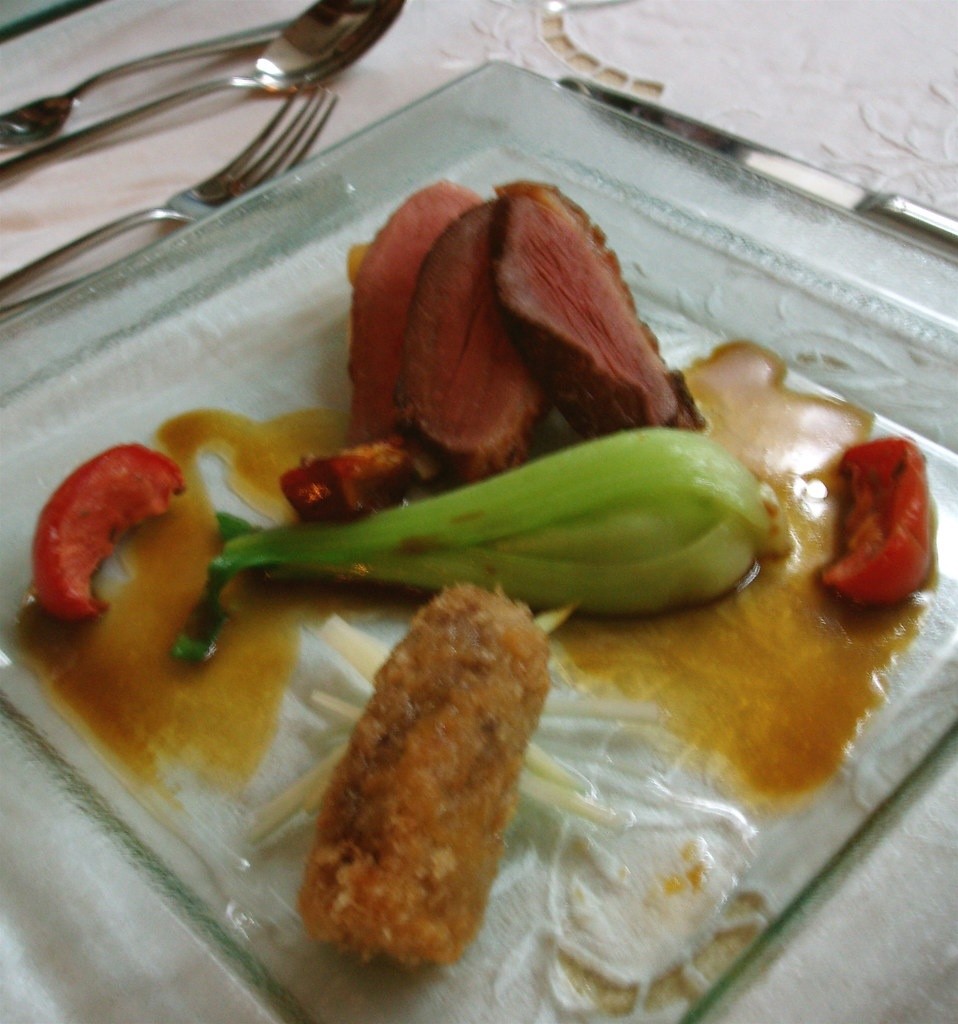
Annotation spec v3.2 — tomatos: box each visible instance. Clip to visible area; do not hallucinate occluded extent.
[31,442,186,621]
[820,435,935,602]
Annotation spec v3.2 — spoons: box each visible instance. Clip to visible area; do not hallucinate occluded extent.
[0,0,405,203]
[0,19,318,146]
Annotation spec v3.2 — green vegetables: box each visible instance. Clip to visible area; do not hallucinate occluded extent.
[173,423,788,664]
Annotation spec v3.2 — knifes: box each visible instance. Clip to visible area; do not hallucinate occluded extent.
[557,76,958,249]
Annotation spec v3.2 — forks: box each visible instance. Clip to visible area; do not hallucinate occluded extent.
[0,86,338,320]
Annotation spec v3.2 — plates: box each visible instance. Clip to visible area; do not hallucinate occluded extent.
[0,62,958,1024]
